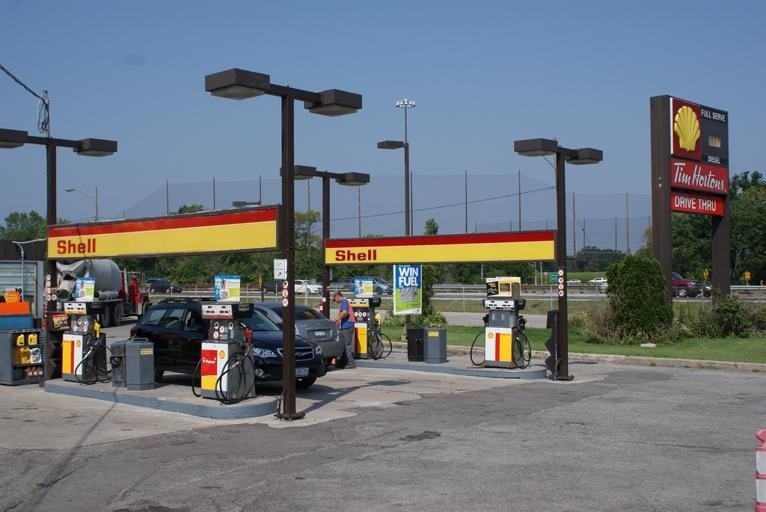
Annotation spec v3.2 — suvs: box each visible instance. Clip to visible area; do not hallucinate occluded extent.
[672,272,702,297]
[350,275,392,294]
[128,296,327,395]
[261,279,323,297]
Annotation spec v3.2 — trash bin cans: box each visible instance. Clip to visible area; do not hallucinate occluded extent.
[405,323,424,362]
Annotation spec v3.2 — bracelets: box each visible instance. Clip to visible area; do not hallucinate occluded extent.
[335,318,337,322]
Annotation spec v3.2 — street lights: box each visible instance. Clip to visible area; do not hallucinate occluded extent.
[64,185,99,223]
[278,165,370,326]
[0,128,118,309]
[203,67,363,424]
[396,97,415,142]
[230,199,264,302]
[375,140,411,238]
[511,137,604,382]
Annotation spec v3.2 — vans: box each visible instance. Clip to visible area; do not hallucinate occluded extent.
[146,278,181,293]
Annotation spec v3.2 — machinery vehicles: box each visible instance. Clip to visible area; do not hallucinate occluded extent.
[54,258,152,328]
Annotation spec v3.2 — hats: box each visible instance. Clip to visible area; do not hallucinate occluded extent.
[333,291,339,302]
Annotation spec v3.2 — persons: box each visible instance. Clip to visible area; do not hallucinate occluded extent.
[331,290,357,369]
[77,281,86,297]
[219,279,230,299]
[358,282,367,294]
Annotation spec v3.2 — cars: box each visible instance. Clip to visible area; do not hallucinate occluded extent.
[567,279,585,286]
[699,281,713,297]
[588,276,608,286]
[249,302,345,369]
